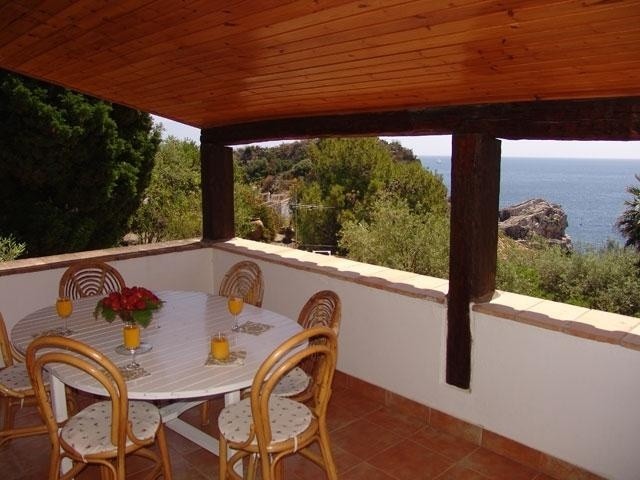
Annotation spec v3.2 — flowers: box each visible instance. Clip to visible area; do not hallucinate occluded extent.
[95,287,165,327]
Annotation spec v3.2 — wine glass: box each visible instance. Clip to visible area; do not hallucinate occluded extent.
[122,322,141,371]
[227,294,244,332]
[55,296,75,335]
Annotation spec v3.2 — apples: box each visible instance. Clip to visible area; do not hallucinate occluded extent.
[101,286,159,313]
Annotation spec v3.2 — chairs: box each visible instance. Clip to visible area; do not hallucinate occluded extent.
[201,260,265,425]
[59,259,126,403]
[242,291,342,401]
[219,327,338,480]
[0,312,79,448]
[27,337,172,480]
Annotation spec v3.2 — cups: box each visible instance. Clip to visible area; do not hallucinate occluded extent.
[209,333,232,363]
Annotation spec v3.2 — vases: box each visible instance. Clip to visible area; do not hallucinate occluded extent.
[116,322,153,357]
